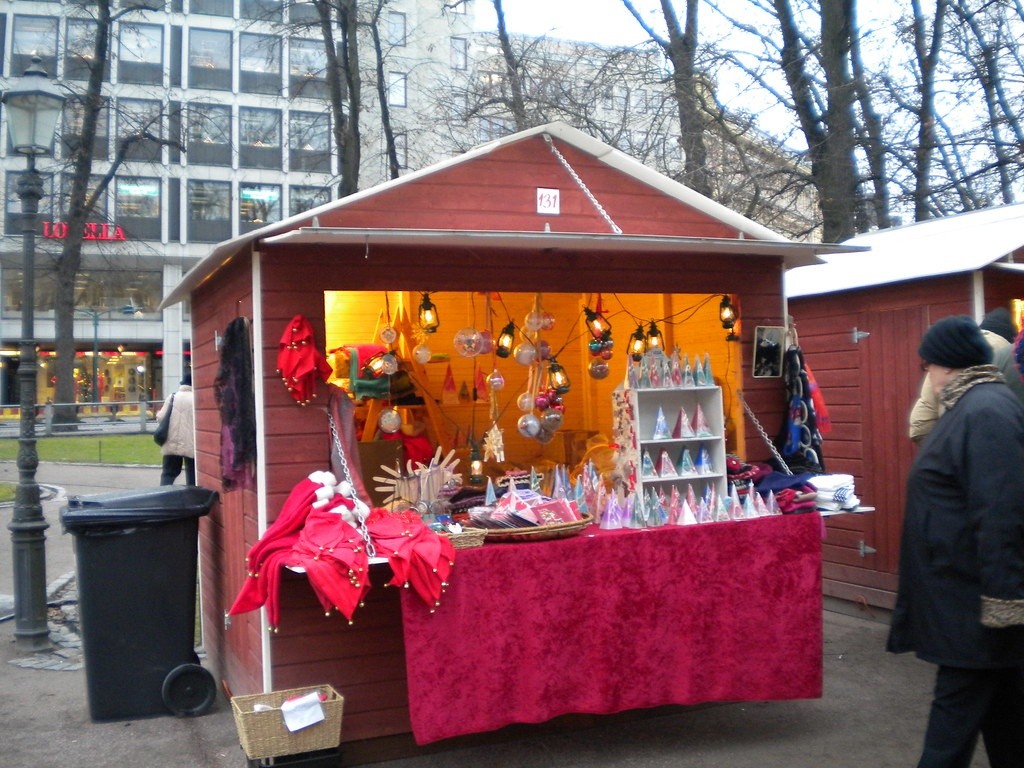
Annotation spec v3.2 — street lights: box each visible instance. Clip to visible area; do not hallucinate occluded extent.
[72,304,138,414]
[1,49,74,652]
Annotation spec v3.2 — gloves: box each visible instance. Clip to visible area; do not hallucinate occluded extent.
[984,624,1024,663]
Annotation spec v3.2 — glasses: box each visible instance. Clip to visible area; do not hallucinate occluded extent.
[919,360,933,370]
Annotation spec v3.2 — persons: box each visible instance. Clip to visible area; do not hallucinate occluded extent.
[156,374,196,486]
[50,376,56,384]
[91,367,105,402]
[886,308,1024,768]
[73,368,84,402]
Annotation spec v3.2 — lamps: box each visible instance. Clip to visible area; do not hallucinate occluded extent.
[494,319,515,359]
[719,294,738,329]
[626,322,645,362]
[548,356,571,394]
[582,305,612,344]
[647,321,665,353]
[418,290,440,332]
[362,349,397,380]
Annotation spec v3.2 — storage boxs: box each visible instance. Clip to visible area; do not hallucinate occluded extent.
[241,745,341,768]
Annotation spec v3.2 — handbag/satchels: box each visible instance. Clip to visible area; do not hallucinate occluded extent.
[153,393,176,446]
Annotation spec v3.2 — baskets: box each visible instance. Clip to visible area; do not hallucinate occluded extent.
[438,526,488,549]
[230,683,345,760]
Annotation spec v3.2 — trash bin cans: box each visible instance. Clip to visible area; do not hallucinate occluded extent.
[58,483,219,723]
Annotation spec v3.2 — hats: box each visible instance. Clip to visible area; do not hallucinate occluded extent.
[180,374,191,386]
[917,315,993,369]
[981,307,1016,345]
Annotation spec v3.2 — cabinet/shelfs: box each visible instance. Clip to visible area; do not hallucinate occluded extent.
[610,385,728,515]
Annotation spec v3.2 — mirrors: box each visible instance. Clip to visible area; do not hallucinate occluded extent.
[752,325,783,378]
